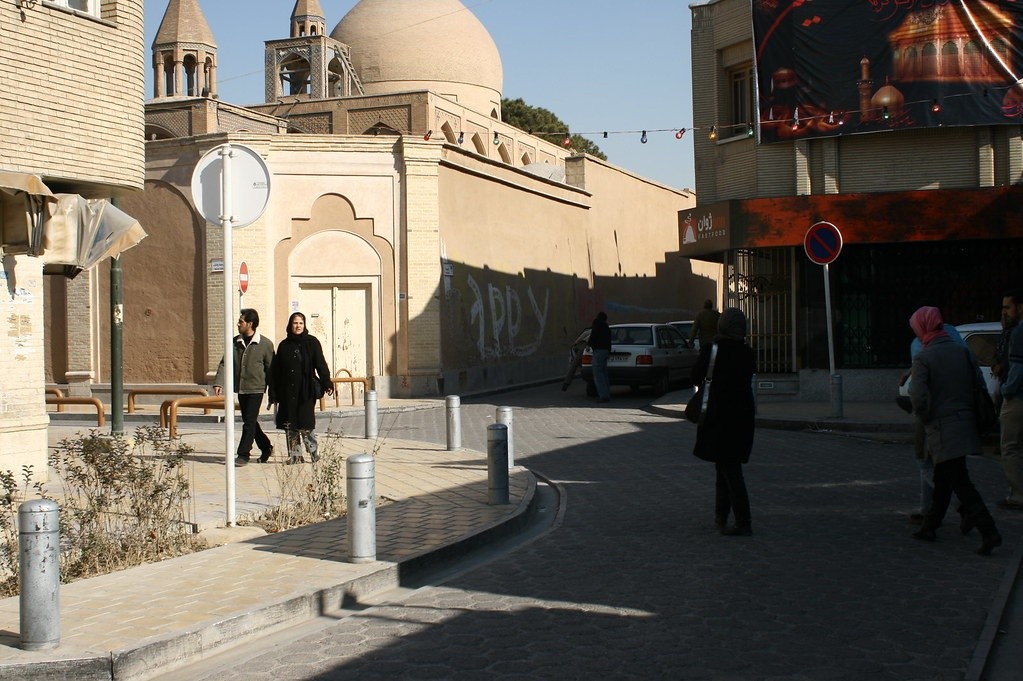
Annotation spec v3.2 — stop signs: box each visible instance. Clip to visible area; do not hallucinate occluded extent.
[238,261,250,294]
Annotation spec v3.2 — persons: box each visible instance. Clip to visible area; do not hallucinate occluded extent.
[992,292,1023,509]
[910,306,1003,555]
[688,300,719,385]
[690,309,757,535]
[268,312,334,465]
[213,308,275,467]
[586,312,612,403]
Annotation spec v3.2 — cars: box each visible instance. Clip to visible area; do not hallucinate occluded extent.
[666,320,702,349]
[895,322,1007,415]
[578,322,701,395]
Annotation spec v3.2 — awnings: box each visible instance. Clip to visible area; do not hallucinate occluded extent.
[44,192,149,278]
[0,168,59,256]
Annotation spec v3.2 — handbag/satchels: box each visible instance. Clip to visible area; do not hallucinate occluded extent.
[311,376,325,400]
[685,380,713,426]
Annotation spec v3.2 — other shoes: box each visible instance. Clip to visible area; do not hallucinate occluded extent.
[960,518,974,533]
[912,529,936,541]
[235,455,248,467]
[999,500,1023,511]
[702,521,722,530]
[722,525,753,536]
[283,455,305,466]
[257,445,273,463]
[312,447,320,463]
[910,513,925,523]
[977,536,1001,556]
